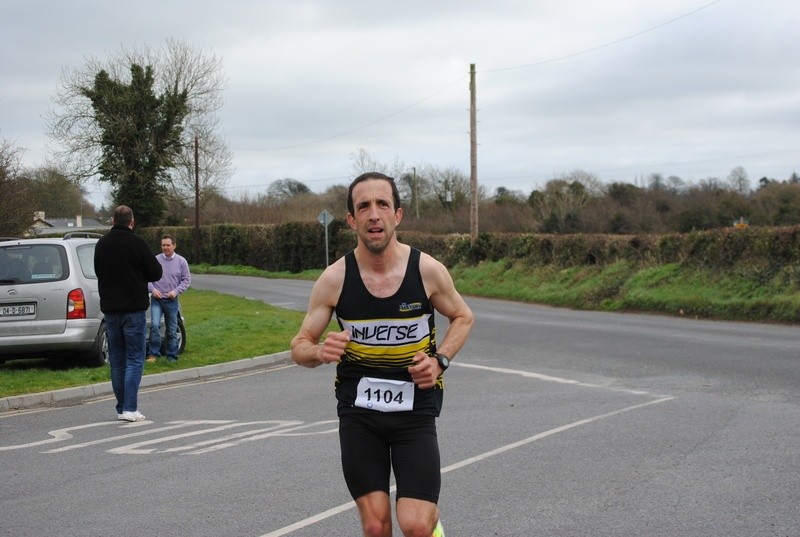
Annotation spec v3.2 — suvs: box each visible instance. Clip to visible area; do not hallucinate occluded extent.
[0,232,187,367]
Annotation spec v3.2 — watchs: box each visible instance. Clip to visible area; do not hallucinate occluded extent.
[428,352,449,370]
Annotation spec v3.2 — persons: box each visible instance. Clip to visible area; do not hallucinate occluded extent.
[290,172,474,536]
[146,233,191,363]
[93,205,164,422]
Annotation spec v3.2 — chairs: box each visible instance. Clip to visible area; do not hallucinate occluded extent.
[32,262,54,274]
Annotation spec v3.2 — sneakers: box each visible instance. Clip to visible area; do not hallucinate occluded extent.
[122,410,145,422]
[118,413,123,419]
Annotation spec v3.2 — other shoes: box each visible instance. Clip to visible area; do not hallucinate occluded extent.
[146,355,156,363]
[432,520,444,537]
